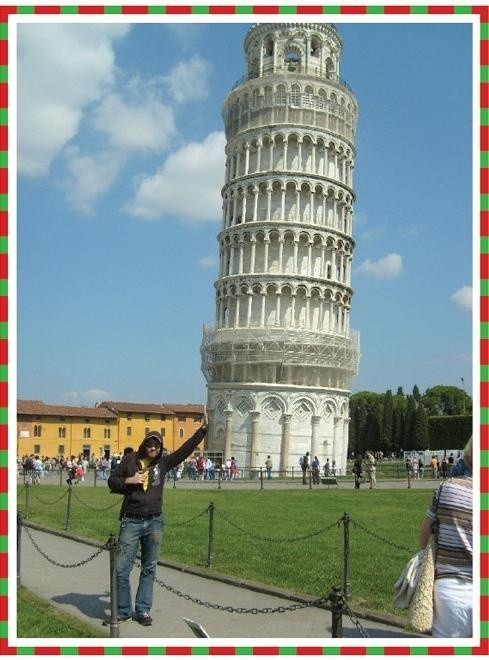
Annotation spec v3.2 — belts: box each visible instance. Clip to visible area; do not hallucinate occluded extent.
[123,512,161,521]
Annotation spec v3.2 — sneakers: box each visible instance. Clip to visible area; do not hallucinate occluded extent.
[103,616,132,626]
[136,612,153,626]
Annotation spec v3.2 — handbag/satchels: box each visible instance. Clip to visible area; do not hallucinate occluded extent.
[408,542,438,633]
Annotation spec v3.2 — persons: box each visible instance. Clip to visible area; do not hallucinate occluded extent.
[347,449,470,488]
[17,448,134,486]
[165,456,239,482]
[265,451,337,485]
[415,432,473,637]
[101,403,210,630]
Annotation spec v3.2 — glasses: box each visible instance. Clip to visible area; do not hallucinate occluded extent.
[144,439,162,449]
